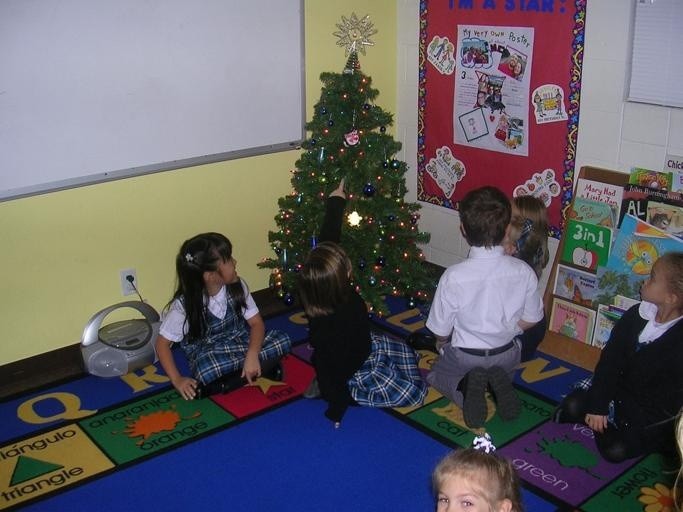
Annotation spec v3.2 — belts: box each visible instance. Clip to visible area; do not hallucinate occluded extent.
[460,337,517,356]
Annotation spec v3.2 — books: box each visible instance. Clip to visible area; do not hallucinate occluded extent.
[548,176,681,353]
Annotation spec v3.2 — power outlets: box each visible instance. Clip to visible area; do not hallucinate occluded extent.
[120,270,141,295]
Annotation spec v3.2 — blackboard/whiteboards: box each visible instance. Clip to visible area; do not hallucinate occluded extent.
[623,0,683,110]
[0,0,306,203]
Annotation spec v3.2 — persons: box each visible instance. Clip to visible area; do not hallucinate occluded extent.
[154,231,291,401]
[554,250,682,464]
[295,177,428,429]
[430,427,524,512]
[424,184,544,428]
[500,195,550,358]
[462,45,523,108]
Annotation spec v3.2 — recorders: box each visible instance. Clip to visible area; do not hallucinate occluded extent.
[81,301,174,378]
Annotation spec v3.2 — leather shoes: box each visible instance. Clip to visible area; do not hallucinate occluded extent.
[486,365,522,421]
[411,335,439,354]
[553,406,577,426]
[263,360,283,382]
[462,366,489,430]
[194,378,220,401]
